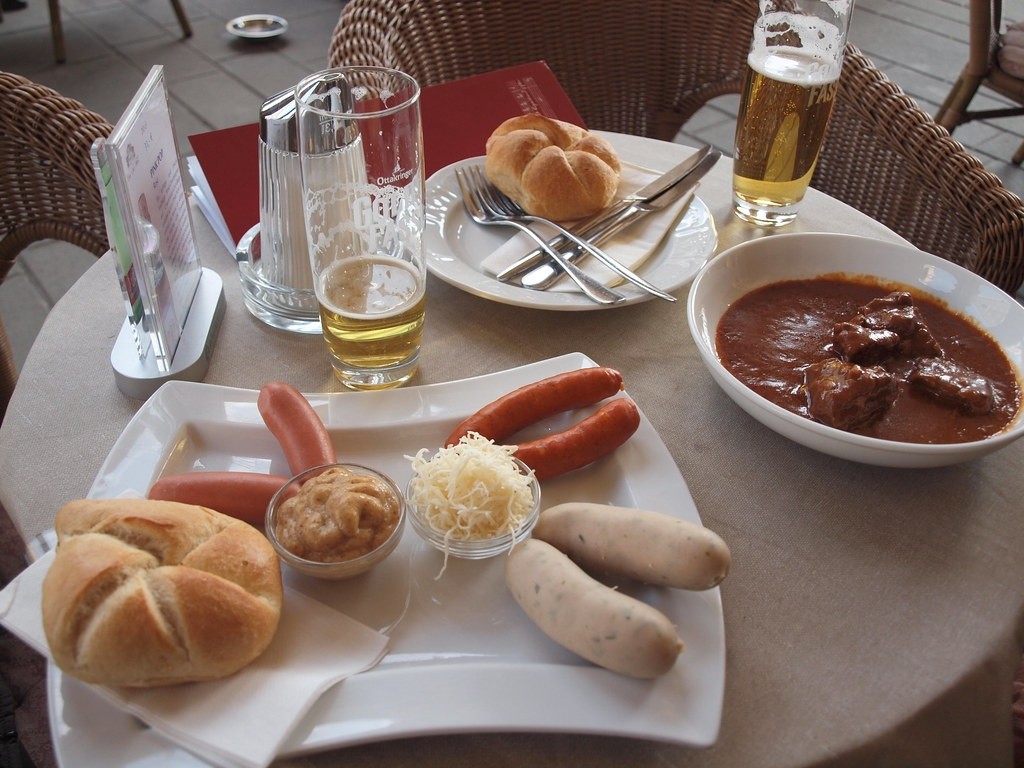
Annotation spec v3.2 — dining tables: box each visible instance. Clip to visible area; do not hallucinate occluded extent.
[0,130,1024,768]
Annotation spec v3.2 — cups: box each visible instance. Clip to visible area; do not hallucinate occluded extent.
[732,0,854,227]
[294,65,429,390]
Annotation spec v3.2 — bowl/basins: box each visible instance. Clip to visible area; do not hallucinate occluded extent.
[264,459,405,585]
[407,446,541,562]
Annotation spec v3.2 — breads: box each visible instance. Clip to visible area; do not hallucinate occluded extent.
[485,113,620,218]
[42,499,284,688]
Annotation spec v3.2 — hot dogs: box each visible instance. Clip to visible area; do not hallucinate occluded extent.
[442,368,638,478]
[148,383,335,513]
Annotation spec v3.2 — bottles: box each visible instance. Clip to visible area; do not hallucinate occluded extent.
[257,74,370,301]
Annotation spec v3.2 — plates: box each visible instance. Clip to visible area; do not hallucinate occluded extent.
[48,345,727,767]
[423,152,720,311]
[687,229,1024,470]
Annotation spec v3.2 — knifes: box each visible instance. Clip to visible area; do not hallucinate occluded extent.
[520,148,726,289]
[497,143,714,283]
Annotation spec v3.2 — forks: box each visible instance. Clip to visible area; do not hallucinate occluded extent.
[454,162,627,307]
[470,163,680,304]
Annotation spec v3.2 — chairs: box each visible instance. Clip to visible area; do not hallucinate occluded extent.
[328,0,1023,293]
